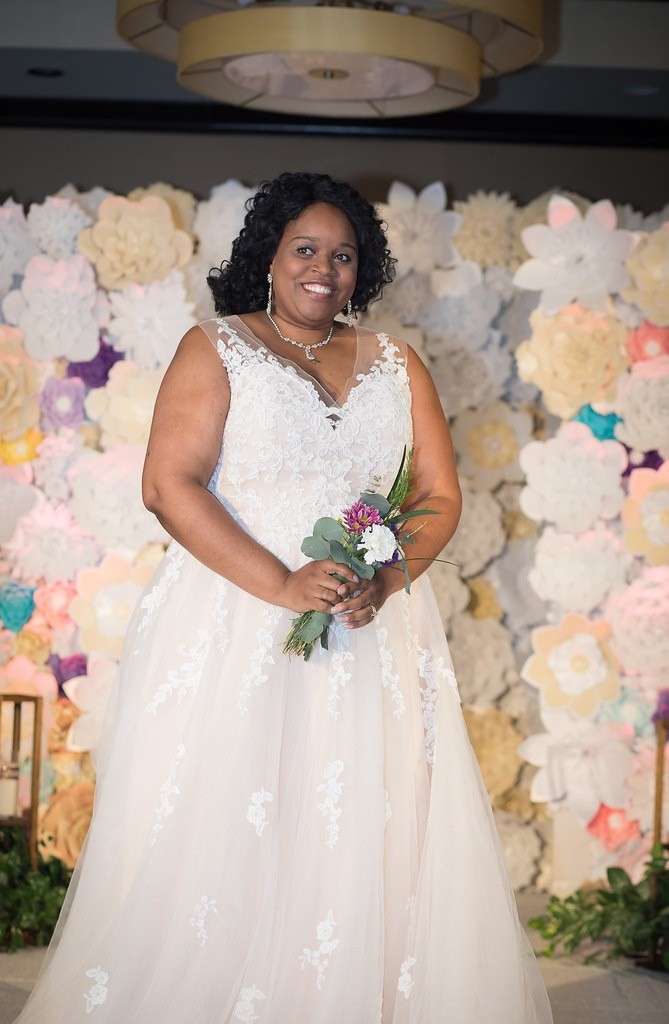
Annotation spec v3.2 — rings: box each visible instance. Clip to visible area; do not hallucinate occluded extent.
[370,604,377,617]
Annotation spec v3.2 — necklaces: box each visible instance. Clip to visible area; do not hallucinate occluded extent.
[266,308,333,360]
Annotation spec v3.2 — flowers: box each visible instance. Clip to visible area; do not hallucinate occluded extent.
[280,441,461,664]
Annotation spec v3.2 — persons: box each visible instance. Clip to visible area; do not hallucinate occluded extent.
[17,172,554,1023]
